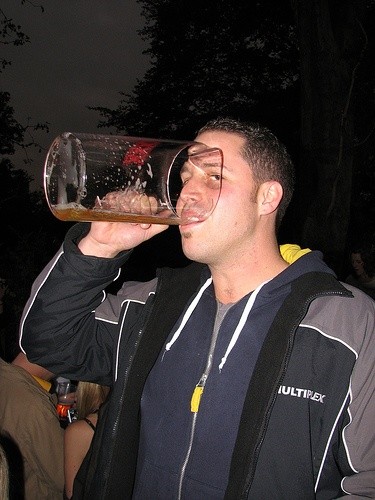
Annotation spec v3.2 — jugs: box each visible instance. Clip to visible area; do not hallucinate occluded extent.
[42,130,224,226]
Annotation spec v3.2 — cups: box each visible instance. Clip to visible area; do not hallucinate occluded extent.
[56,381,78,406]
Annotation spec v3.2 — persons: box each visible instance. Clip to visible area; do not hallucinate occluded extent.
[49,376,77,430]
[344,247,375,301]
[18,117,375,500]
[0,352,64,500]
[62,380,109,500]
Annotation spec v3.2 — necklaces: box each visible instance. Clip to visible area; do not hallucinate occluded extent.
[90,409,98,413]
